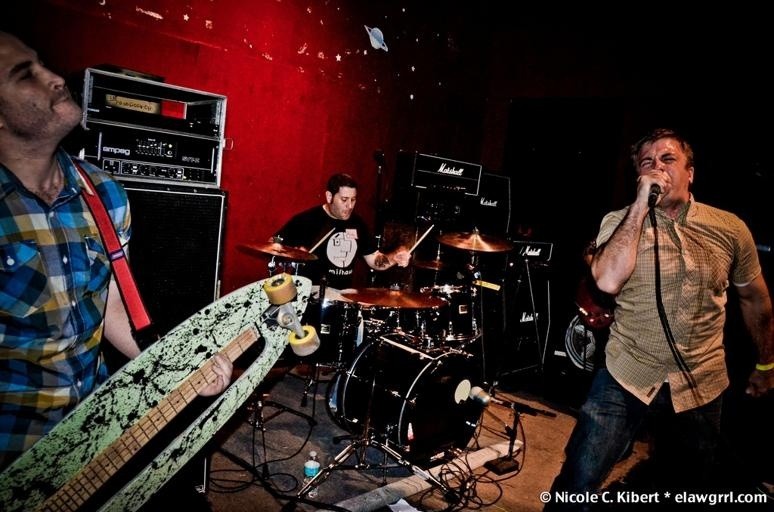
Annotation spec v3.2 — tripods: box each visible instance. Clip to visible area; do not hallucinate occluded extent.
[278,316,464,511]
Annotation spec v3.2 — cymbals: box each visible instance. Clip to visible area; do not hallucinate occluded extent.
[342,286,447,309]
[437,228,513,253]
[239,238,318,262]
[410,259,443,271]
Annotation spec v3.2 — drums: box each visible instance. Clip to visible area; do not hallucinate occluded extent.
[362,303,400,337]
[337,330,485,464]
[305,285,364,361]
[564,310,604,373]
[420,283,477,343]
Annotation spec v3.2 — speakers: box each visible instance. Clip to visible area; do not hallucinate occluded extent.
[97,191,229,497]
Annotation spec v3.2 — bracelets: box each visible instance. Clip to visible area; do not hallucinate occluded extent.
[756,362,774,370]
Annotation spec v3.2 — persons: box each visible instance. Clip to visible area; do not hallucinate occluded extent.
[542,126,772,511]
[267,170,414,411]
[0,28,235,509]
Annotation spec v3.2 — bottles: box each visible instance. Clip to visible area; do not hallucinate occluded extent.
[303,450,320,498]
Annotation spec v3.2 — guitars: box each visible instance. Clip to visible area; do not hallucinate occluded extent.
[33,323,263,511]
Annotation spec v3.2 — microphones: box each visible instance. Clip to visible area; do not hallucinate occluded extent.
[646,177,664,210]
[469,384,493,411]
